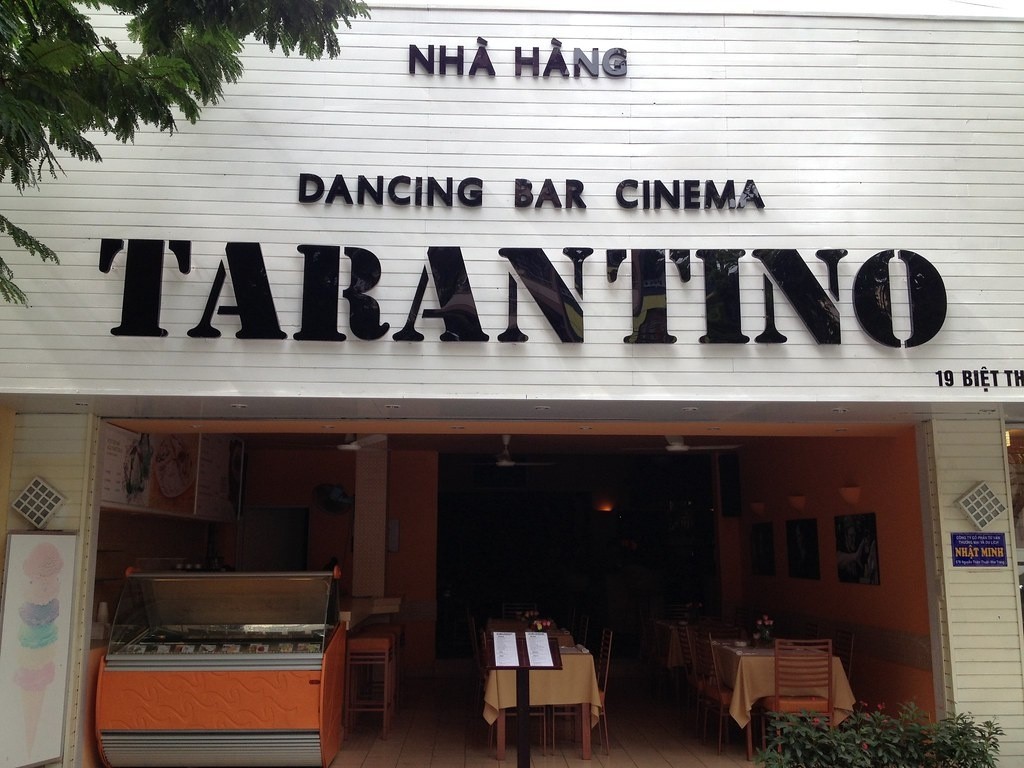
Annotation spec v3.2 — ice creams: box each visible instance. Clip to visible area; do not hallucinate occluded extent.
[13,542,64,759]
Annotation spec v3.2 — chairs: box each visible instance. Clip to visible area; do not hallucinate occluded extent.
[464,587,821,755]
[758,640,833,753]
[826,627,855,681]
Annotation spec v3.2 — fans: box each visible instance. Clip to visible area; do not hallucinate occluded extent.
[277,431,392,452]
[621,433,739,450]
[311,483,350,515]
[469,435,554,467]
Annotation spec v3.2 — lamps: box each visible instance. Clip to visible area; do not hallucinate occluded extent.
[10,475,66,531]
[953,481,1007,530]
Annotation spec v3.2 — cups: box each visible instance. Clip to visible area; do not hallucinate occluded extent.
[96,602,108,622]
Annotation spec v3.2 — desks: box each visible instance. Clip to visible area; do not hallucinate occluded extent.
[482,616,855,761]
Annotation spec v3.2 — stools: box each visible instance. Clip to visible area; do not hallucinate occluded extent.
[343,623,406,741]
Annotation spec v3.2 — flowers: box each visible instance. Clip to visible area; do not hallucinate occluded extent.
[529,619,551,632]
[753,613,773,642]
[685,602,701,611]
[520,609,538,621]
